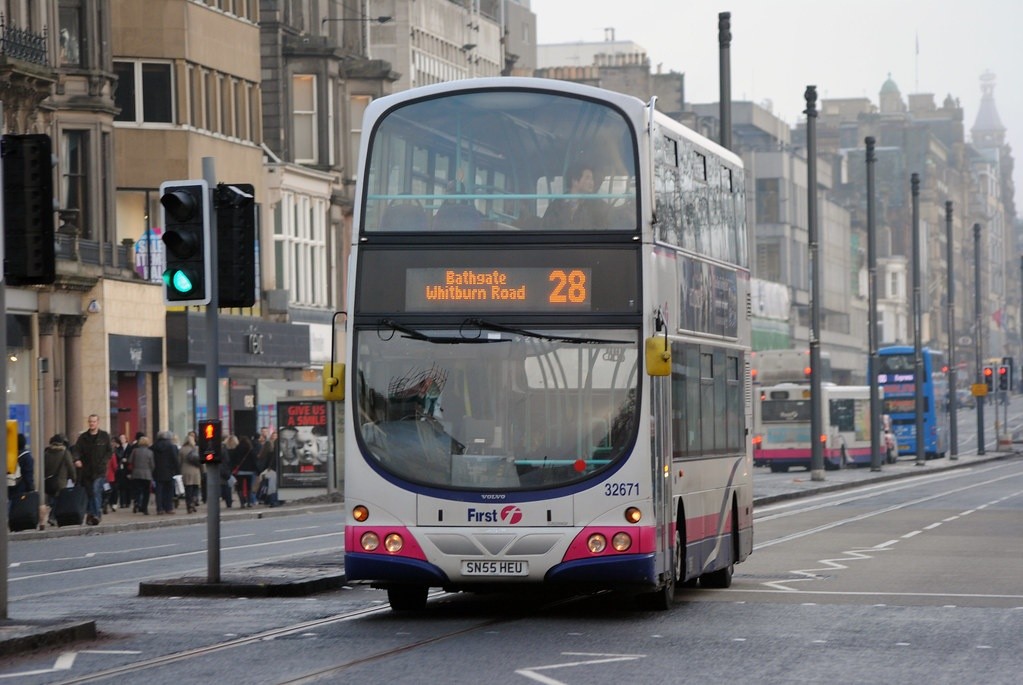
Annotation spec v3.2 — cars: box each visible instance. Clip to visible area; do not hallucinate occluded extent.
[883,415,898,463]
[955,389,975,410]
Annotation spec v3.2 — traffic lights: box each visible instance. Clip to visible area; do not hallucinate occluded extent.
[983,366,994,394]
[198,419,222,465]
[159,179,211,307]
[998,364,1011,393]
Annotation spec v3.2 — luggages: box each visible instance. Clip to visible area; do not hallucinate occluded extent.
[9,489,39,532]
[56,468,88,527]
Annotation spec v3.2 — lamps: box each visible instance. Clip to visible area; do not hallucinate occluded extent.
[120,238,136,247]
[323,16,393,23]
[463,43,476,51]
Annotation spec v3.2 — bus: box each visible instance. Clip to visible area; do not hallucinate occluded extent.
[325,75,754,616]
[752,382,887,473]
[877,346,949,461]
[751,348,833,390]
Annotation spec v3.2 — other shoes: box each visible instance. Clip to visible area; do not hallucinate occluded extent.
[111,504,118,512]
[86,514,99,525]
[103,503,110,514]
[120,503,131,508]
[165,509,176,514]
[187,501,280,511]
[157,510,164,514]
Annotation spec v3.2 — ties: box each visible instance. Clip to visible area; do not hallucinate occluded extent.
[426,397,438,421]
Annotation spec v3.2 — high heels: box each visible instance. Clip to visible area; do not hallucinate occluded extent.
[140,508,148,515]
[133,502,138,513]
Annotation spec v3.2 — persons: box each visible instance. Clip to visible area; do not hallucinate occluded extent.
[74,414,113,525]
[295,430,319,465]
[390,375,437,421]
[102,431,207,515]
[430,180,480,230]
[221,427,287,509]
[310,425,328,465]
[44,434,77,527]
[538,163,610,226]
[7,433,34,532]
[279,426,298,467]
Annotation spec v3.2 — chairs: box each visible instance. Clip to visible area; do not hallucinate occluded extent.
[381,192,429,230]
[431,192,480,231]
[611,192,636,228]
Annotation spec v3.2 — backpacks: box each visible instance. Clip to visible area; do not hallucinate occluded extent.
[7,451,30,486]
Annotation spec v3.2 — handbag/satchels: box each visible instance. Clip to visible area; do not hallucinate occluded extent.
[172,475,185,495]
[232,465,241,474]
[45,475,59,494]
[187,449,201,466]
